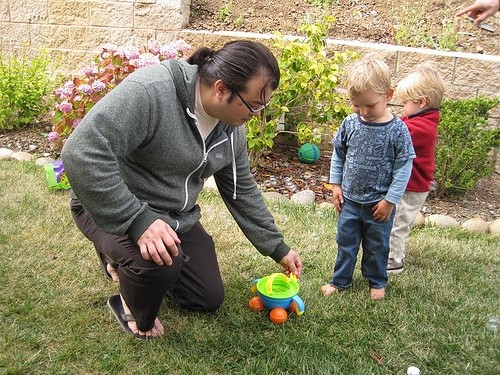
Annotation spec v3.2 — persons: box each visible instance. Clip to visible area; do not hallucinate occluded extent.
[60,39,302,338]
[319,57,445,300]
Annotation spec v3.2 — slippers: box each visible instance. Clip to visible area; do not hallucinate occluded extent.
[107,295,164,340]
[93,241,120,284]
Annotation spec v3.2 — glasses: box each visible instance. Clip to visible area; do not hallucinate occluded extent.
[236,91,270,116]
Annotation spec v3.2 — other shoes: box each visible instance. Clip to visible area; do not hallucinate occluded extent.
[386,258,403,274]
